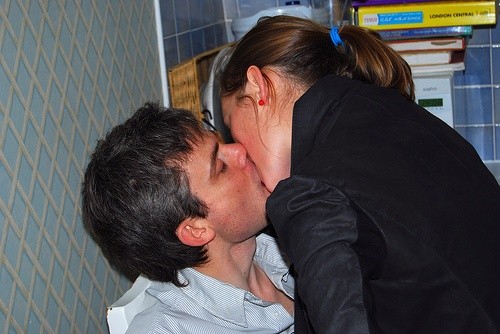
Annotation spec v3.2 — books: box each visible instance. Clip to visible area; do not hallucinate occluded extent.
[351,1,497,129]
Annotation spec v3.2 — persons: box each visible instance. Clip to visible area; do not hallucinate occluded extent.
[221,15,499,334]
[83,101,295,333]
